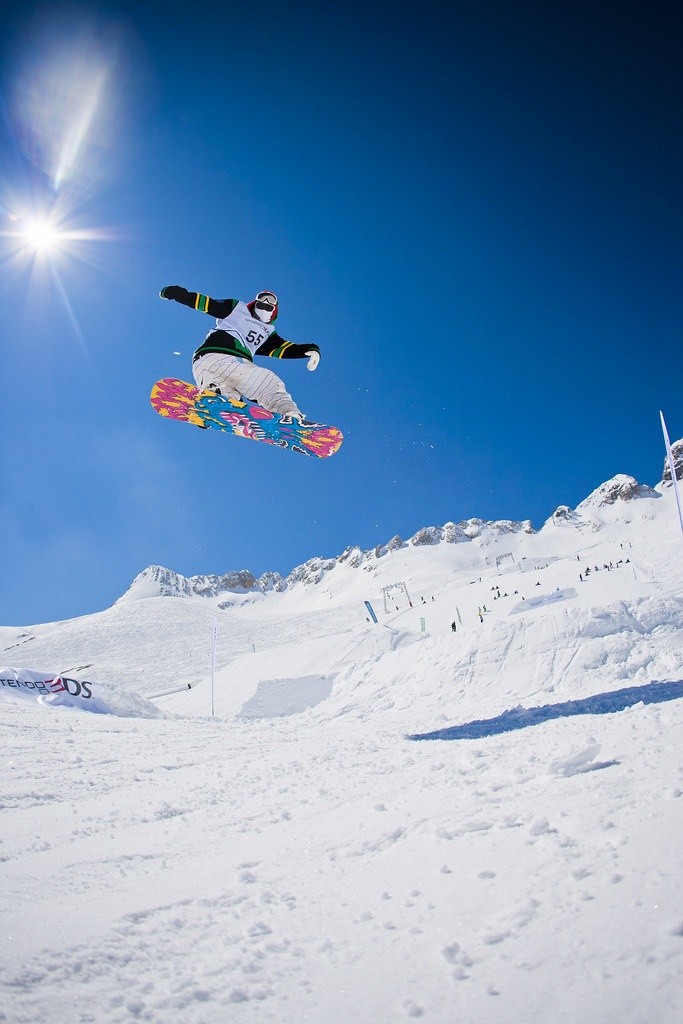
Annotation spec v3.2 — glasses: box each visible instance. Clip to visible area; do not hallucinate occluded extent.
[255,302,275,312]
[256,292,278,305]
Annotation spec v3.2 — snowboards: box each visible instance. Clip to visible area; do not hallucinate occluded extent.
[149,377,345,460]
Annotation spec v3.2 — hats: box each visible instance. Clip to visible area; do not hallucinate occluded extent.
[247,289,277,319]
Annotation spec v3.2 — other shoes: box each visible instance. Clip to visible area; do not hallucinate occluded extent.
[196,383,220,430]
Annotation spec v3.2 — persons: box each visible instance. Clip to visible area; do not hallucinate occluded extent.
[159,286,320,418]
[537,582,539,585]
[579,559,630,581]
[490,586,518,599]
[577,556,580,561]
[421,596,425,604]
[620,543,622,548]
[478,605,486,622]
[452,621,456,632]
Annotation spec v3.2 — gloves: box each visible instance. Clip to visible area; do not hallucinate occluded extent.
[159,285,180,300]
[304,351,320,371]
